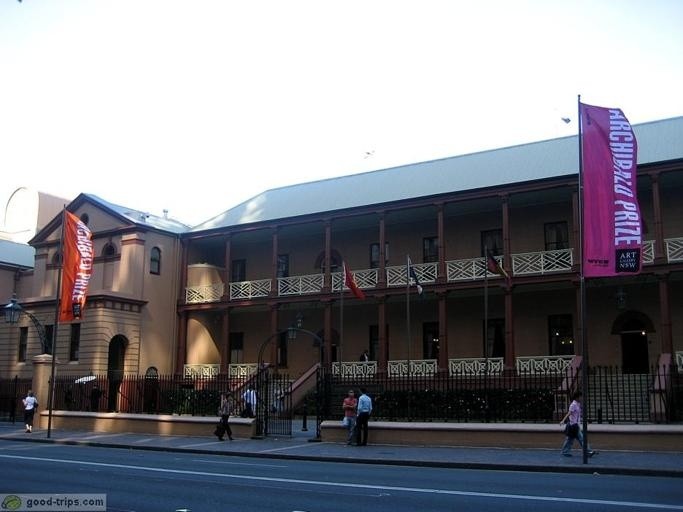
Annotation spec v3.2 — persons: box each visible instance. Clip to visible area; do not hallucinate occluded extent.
[62,384,73,410]
[239,384,260,418]
[217,393,235,441]
[361,351,369,361]
[560,391,596,457]
[356,387,373,446]
[91,385,101,411]
[340,389,357,445]
[22,389,38,433]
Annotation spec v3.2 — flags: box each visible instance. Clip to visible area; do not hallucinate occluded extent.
[344,263,365,301]
[487,248,513,287]
[409,258,424,296]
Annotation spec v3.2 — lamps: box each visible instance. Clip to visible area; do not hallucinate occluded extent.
[296,312,304,328]
[5,292,22,323]
[288,327,297,341]
[616,285,628,310]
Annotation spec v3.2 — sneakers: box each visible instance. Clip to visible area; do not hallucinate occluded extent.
[589,451,595,458]
[218,437,234,440]
[24,428,31,433]
[348,440,367,446]
[563,454,571,457]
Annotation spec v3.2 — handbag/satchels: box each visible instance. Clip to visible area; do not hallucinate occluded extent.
[34,402,39,408]
[214,423,225,436]
[564,422,579,438]
